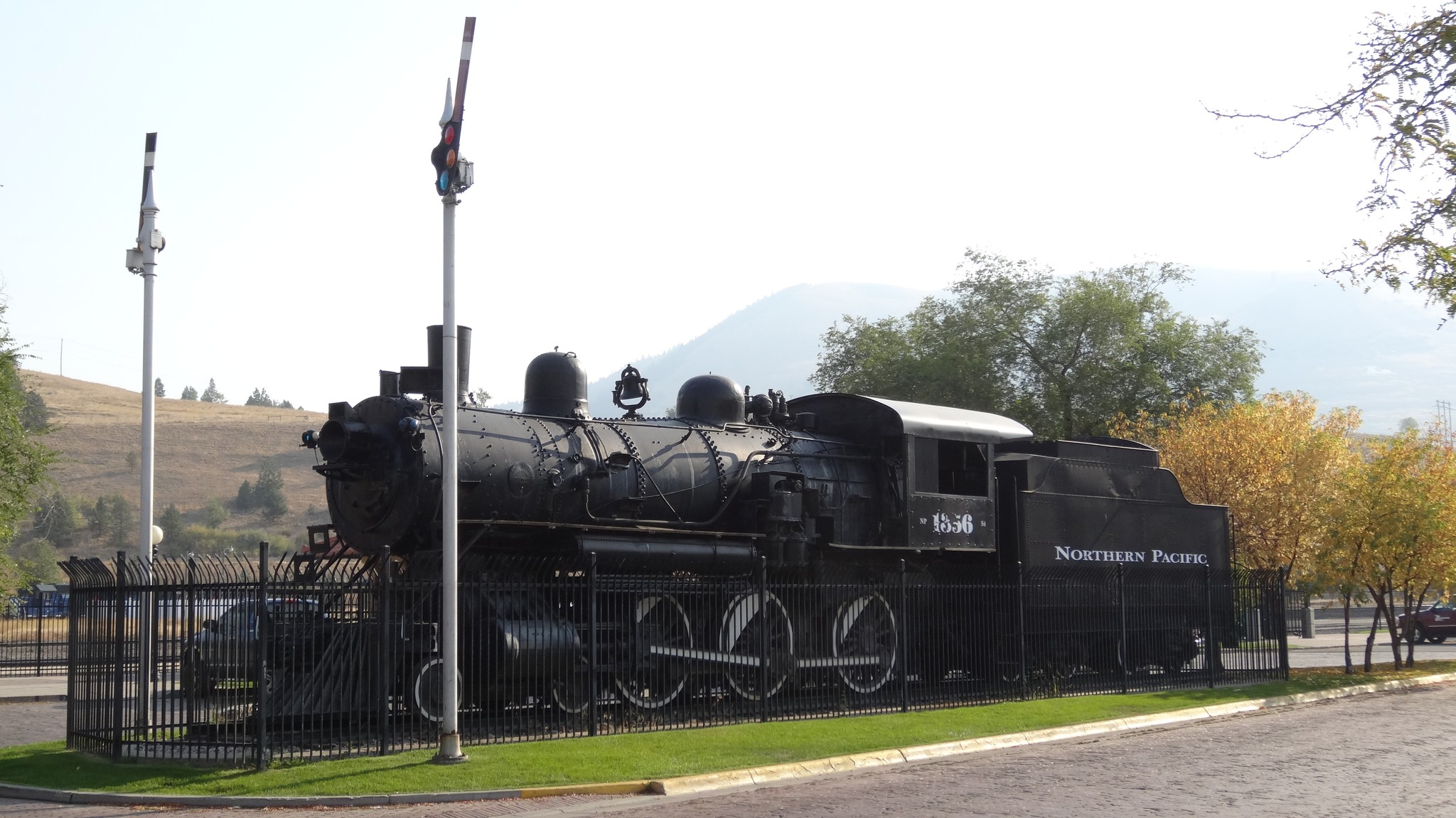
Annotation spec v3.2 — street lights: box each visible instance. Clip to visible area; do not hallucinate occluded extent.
[151,525,164,683]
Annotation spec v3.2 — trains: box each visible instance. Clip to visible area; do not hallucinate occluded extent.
[297,324,1243,721]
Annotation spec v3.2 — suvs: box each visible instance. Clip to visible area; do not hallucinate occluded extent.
[180,599,328,698]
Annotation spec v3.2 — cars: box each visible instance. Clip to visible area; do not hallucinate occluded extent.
[1390,595,1456,643]
